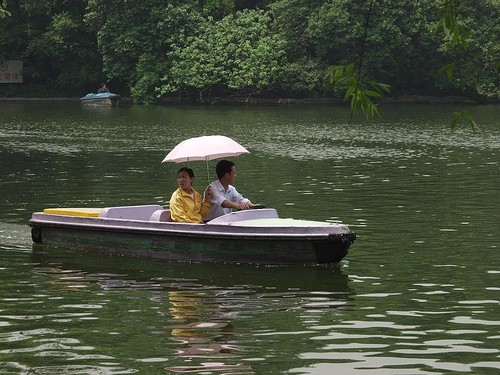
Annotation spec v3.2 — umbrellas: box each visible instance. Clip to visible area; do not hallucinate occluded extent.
[161,135,252,186]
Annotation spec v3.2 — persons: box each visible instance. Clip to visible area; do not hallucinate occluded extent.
[98,82,110,93]
[201,159,255,223]
[170,167,214,223]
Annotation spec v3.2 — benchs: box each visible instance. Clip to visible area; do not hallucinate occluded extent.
[103,204,164,223]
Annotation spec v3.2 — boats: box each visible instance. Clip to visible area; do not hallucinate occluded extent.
[27,205,356,263]
[80,93,120,107]
